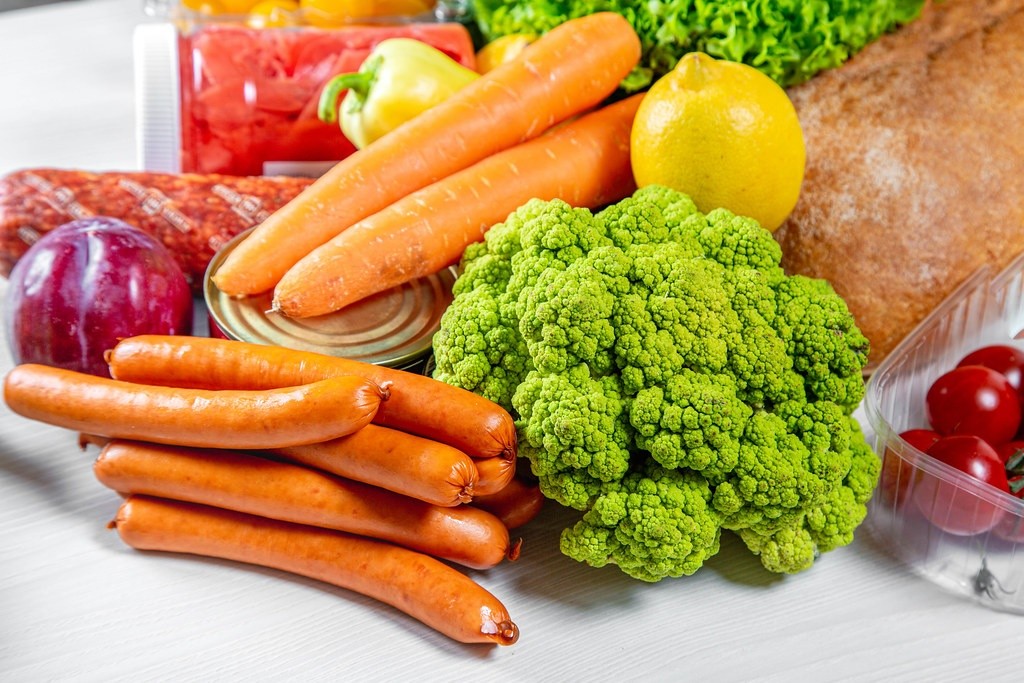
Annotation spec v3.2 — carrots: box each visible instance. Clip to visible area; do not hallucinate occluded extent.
[210,12,649,318]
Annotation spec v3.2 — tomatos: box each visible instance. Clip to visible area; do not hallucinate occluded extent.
[879,345,1024,543]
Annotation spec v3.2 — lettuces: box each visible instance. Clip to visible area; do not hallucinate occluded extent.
[469,0,926,91]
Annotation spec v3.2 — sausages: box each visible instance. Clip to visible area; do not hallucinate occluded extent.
[2,336,541,645]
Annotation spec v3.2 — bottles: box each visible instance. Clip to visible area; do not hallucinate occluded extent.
[133,22,477,177]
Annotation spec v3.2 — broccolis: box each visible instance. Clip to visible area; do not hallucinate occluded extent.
[430,186,880,583]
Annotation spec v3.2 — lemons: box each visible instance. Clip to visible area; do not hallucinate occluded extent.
[630,51,805,235]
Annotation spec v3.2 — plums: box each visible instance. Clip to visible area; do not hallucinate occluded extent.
[3,217,191,390]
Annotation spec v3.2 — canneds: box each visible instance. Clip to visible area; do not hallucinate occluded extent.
[202,222,458,376]
